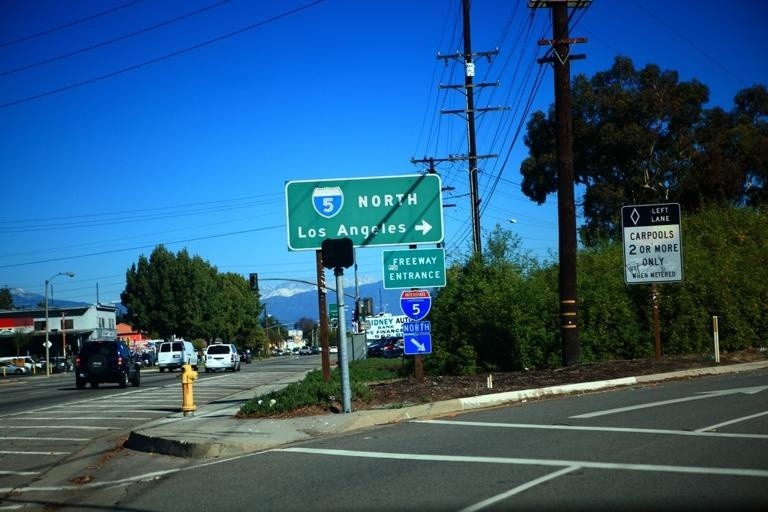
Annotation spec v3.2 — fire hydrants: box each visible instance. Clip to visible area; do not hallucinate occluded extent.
[182,363,198,416]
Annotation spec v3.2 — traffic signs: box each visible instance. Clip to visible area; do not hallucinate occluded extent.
[404,322,431,354]
[383,249,446,289]
[285,174,443,251]
[401,289,431,321]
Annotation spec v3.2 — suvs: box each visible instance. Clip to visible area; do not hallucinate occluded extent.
[76,340,140,390]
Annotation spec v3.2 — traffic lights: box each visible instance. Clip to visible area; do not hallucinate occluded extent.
[249,272,258,292]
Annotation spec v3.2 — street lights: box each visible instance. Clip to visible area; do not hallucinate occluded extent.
[621,202,684,285]
[45,272,75,376]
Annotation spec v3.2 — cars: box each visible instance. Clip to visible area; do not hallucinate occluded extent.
[369,336,404,358]
[241,351,252,364]
[0,356,42,375]
[272,346,319,355]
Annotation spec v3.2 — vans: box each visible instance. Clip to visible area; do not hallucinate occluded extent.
[204,344,240,371]
[158,341,198,372]
